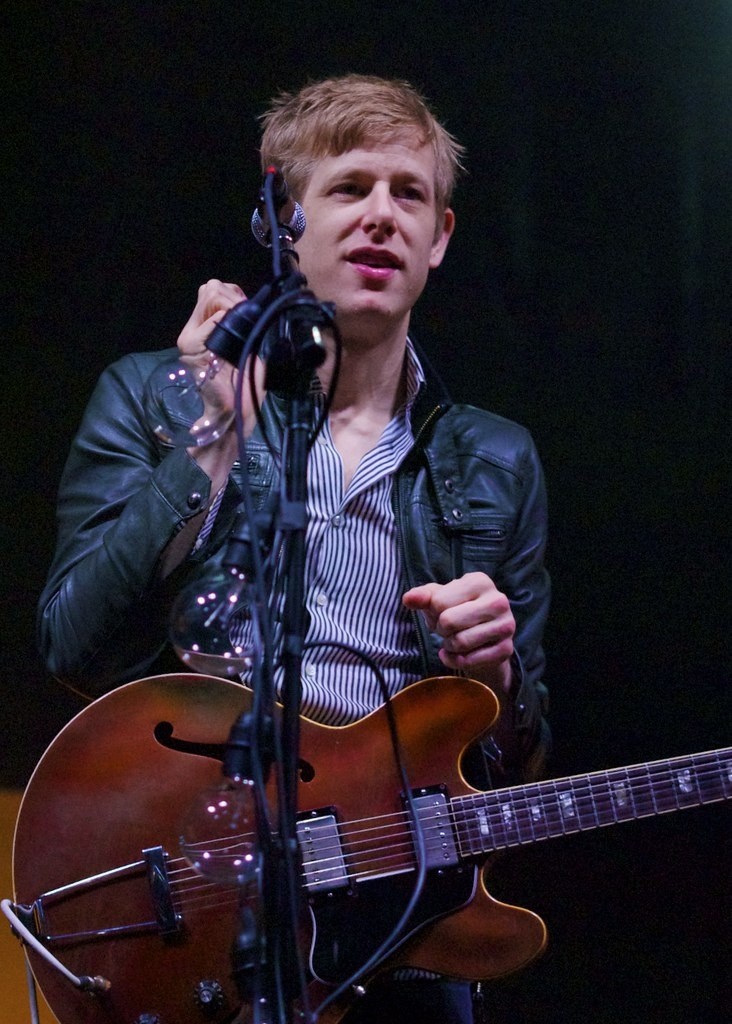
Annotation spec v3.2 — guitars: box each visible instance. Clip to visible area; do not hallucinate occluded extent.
[9,671,732,1024]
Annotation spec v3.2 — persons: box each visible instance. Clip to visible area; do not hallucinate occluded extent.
[32,74,554,1024]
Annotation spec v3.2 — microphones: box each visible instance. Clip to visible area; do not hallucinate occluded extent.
[251,164,306,248]
